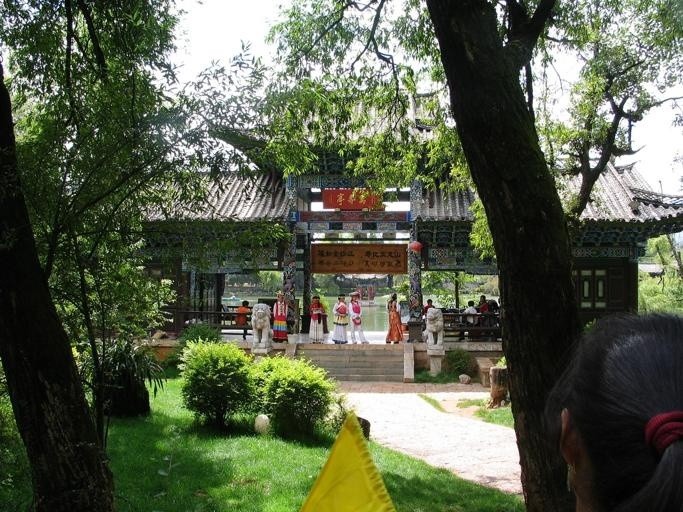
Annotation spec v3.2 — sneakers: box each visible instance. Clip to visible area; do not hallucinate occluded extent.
[335,341,347,344]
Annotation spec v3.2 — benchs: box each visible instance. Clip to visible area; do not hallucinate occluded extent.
[175,306,254,336]
[434,307,501,342]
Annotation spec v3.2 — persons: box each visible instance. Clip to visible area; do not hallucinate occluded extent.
[272,290,288,343]
[235,301,249,340]
[541,310,682,512]
[423,299,434,318]
[477,294,486,308]
[331,293,349,344]
[309,295,325,344]
[348,291,368,344]
[385,293,401,343]
[457,301,478,340]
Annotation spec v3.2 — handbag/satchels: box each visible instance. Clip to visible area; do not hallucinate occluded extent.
[354,319,361,325]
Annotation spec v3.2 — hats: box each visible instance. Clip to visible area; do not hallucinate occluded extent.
[349,291,360,296]
